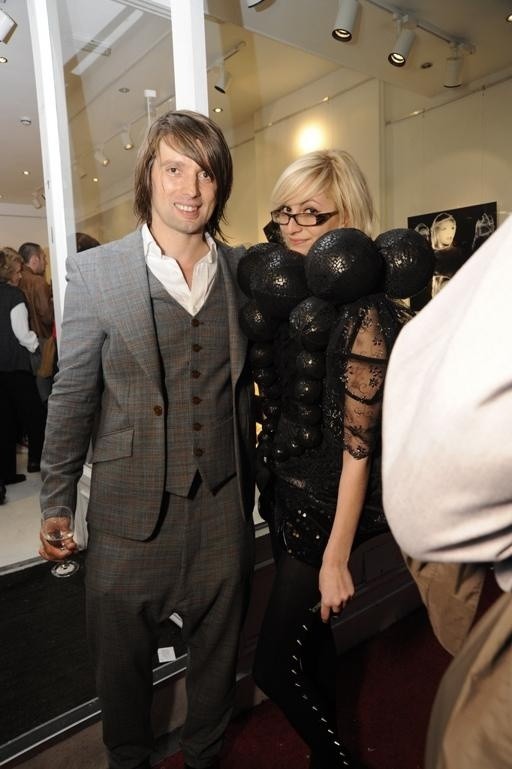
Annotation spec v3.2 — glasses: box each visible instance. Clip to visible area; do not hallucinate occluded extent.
[270,205,340,227]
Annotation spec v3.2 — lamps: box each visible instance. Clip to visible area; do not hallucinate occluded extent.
[31,40,247,209]
[331,0,475,88]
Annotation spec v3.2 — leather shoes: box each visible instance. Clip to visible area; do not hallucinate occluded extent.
[4,453,44,485]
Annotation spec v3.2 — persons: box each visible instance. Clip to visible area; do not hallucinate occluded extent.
[234,150,435,769]
[431,213,457,250]
[18,242,55,474]
[38,111,259,768]
[0,246,39,507]
[382,210,511,768]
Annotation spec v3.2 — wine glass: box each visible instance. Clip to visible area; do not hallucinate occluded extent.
[39,506,79,578]
